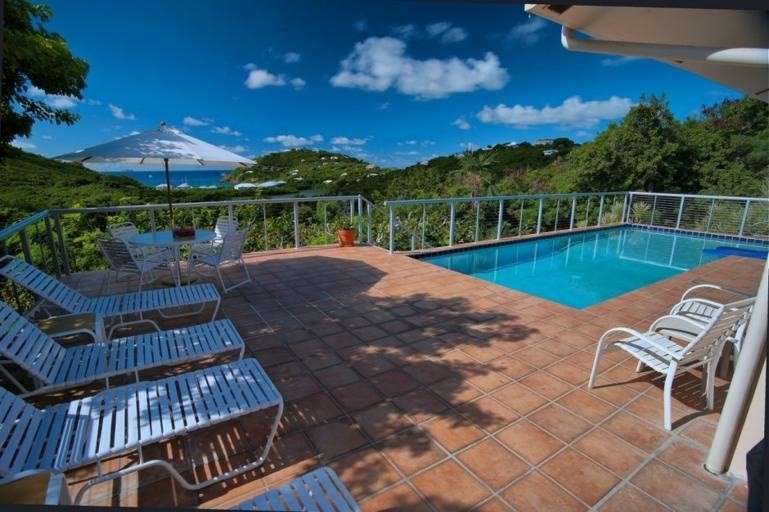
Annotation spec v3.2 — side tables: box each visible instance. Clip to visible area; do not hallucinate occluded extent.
[31,312,107,390]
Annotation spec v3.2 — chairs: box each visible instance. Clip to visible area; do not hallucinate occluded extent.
[96,236,177,320]
[1,254,222,342]
[110,221,167,282]
[635,284,754,395]
[189,215,238,280]
[588,296,755,431]
[1,301,246,401]
[187,227,252,294]
[0,357,284,506]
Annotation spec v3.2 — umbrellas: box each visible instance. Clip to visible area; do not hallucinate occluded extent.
[50,120,257,229]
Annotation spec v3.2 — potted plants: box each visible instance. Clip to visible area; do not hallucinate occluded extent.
[335,218,357,246]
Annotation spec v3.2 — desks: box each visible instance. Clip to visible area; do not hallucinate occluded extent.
[128,229,216,292]
